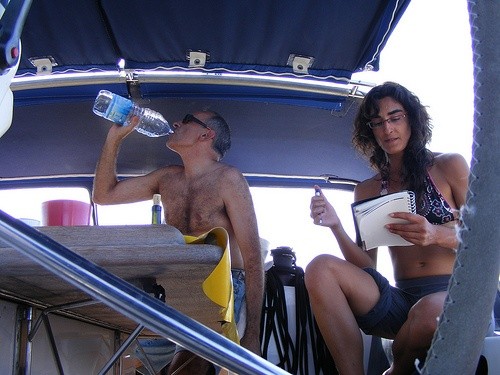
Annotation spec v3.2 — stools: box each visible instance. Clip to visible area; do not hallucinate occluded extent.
[479,332,500,375]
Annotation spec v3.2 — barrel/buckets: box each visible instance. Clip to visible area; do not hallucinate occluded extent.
[42,200,92,226]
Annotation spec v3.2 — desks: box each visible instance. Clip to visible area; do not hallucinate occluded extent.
[0,242,240,375]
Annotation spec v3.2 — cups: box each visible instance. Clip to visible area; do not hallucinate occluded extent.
[271,246,296,266]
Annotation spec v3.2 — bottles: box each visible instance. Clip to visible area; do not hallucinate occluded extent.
[151,194,162,225]
[93,90,173,137]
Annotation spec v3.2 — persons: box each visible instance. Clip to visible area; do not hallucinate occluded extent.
[92,109,265,375]
[304,82,470,375]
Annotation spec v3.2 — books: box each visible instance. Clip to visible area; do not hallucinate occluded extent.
[351,190,417,251]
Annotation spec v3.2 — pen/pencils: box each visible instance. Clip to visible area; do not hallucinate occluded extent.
[316,190,322,222]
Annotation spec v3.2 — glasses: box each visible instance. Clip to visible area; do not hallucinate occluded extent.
[181,113,211,130]
[367,112,407,129]
